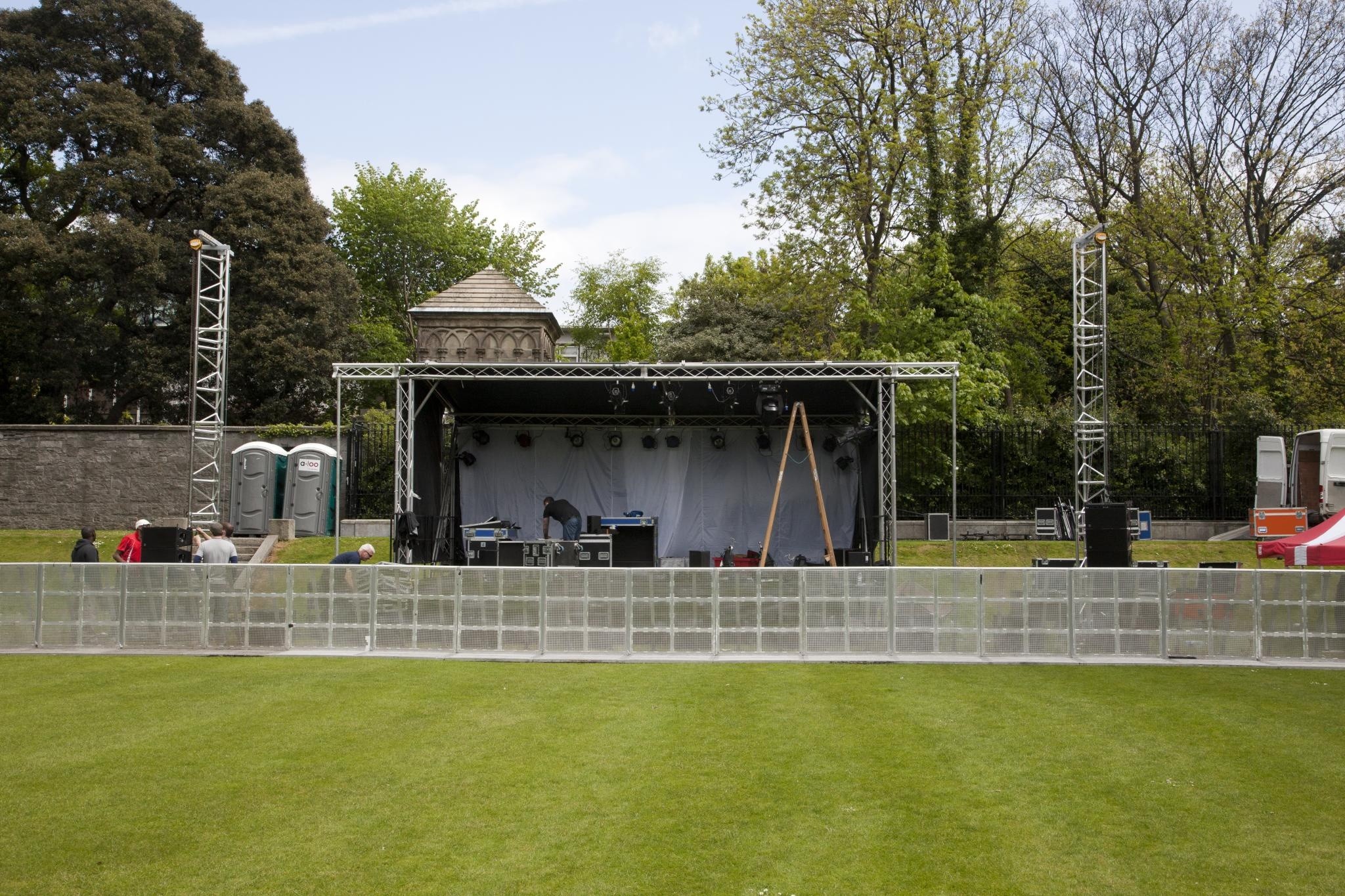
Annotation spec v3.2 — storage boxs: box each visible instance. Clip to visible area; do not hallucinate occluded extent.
[466,533,613,567]
[1249,507,1309,537]
[995,557,1243,627]
[714,557,759,567]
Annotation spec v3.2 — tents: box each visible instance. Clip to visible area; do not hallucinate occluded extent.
[1256,508,1345,652]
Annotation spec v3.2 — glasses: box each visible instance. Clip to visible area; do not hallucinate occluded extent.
[365,551,372,557]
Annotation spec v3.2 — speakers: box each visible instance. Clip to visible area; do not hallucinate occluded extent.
[610,525,657,572]
[141,527,192,562]
[587,515,602,534]
[690,551,710,567]
[1085,503,1132,568]
[848,551,872,566]
[469,540,497,566]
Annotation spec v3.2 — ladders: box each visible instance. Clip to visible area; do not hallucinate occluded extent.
[757,402,836,567]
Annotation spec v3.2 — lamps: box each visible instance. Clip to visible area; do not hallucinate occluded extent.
[665,427,682,448]
[754,428,773,457]
[606,381,631,410]
[707,427,726,449]
[455,451,477,467]
[472,426,489,447]
[515,430,532,447]
[720,380,790,416]
[794,430,854,470]
[659,381,683,408]
[641,435,657,449]
[564,427,584,447]
[602,427,622,451]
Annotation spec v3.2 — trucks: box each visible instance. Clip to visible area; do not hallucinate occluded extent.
[1253,429,1345,528]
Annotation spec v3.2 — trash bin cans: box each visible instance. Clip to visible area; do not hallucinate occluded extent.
[229,440,288,537]
[281,442,344,538]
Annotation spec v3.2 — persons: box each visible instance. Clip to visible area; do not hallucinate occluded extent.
[71,526,108,651]
[112,519,159,649]
[318,543,375,645]
[193,522,238,648]
[542,496,582,542]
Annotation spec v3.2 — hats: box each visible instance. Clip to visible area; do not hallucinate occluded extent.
[135,519,153,529]
